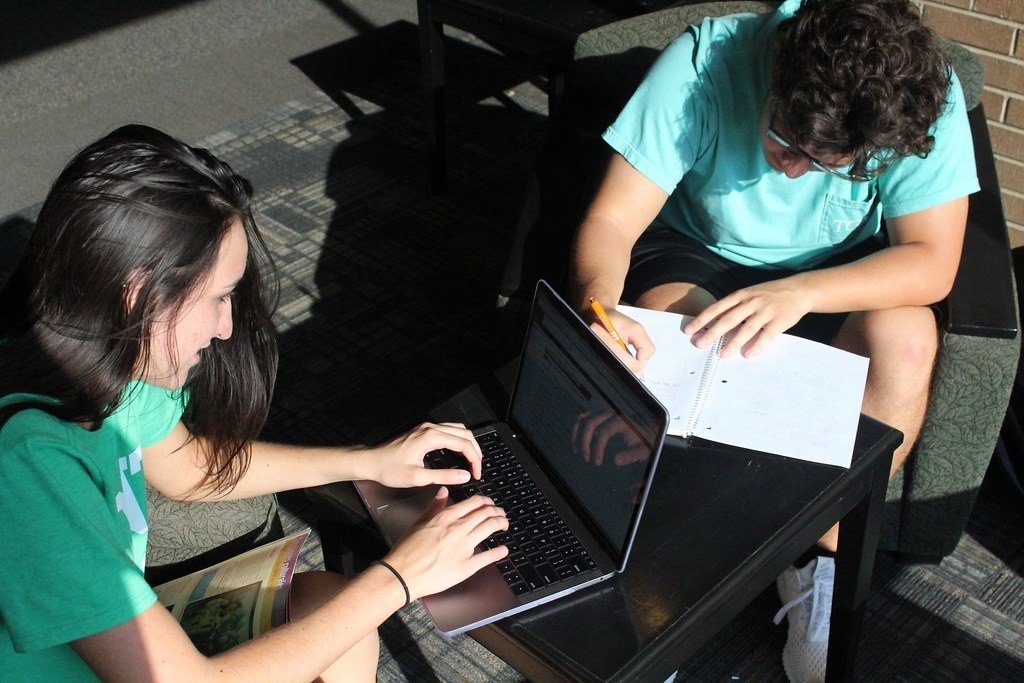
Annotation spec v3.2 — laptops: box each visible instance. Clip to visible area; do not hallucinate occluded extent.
[350,280,671,638]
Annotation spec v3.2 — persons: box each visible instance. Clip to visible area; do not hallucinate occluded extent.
[0,121,509,683]
[569,0,980,683]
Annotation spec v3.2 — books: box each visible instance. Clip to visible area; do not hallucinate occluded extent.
[149,527,312,657]
[611,303,872,470]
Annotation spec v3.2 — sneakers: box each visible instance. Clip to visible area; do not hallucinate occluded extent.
[773,556,836,683]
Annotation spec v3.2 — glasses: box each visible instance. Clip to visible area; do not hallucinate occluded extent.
[766,108,879,182]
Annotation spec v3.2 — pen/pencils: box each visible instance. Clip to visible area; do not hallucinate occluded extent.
[590,296,635,359]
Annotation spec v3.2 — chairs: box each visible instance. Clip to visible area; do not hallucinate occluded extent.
[500,1,1022,567]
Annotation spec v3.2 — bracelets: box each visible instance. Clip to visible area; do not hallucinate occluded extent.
[370,558,411,609]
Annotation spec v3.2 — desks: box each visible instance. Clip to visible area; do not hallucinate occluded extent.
[301,353,903,683]
[416,0,649,194]
